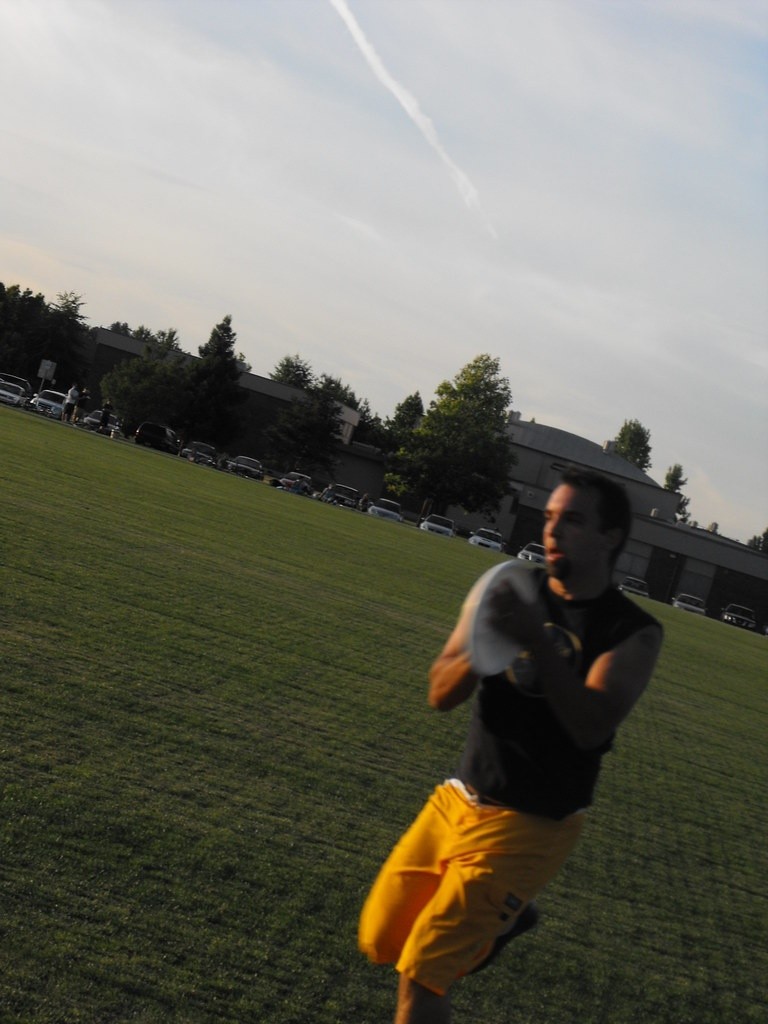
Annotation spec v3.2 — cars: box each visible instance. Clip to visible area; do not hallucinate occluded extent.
[82,410,122,438]
[317,482,361,511]
[182,441,218,468]
[276,471,313,498]
[618,576,651,599]
[518,542,546,566]
[225,456,265,482]
[468,527,506,554]
[366,498,405,525]
[720,604,758,629]
[419,513,458,540]
[0,379,30,409]
[671,593,709,617]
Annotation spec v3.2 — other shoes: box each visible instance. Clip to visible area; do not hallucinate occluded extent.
[466,904,540,974]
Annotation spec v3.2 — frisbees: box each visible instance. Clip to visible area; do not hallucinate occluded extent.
[461,557,545,679]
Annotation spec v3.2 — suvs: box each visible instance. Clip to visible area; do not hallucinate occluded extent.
[31,389,68,422]
[134,422,180,455]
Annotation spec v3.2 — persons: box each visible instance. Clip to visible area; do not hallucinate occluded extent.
[357,472,666,1024]
[177,439,230,469]
[280,476,313,496]
[319,483,336,503]
[61,383,91,424]
[359,491,371,512]
[98,398,113,435]
